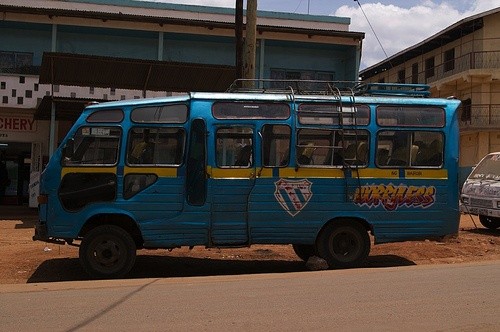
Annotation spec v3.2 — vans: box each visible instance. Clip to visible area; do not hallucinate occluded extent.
[460,151,500,230]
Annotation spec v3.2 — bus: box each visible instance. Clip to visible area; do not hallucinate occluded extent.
[32,78,463,279]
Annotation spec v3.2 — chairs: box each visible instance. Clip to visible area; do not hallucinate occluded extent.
[232,138,444,167]
